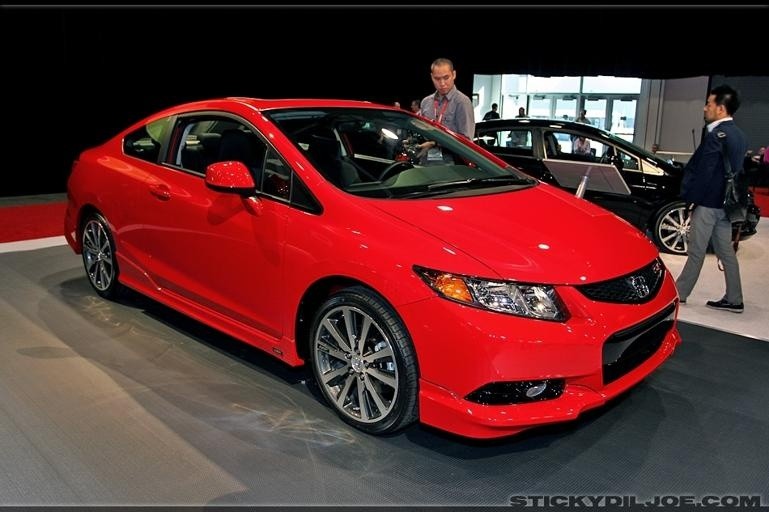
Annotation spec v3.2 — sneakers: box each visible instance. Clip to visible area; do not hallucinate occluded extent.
[679,301,686,304]
[706,295,744,313]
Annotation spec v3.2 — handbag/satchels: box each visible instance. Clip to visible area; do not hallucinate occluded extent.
[724,181,760,235]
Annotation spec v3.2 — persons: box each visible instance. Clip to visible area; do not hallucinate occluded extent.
[651,143,660,156]
[667,83,748,315]
[406,57,478,168]
[760,147,766,155]
[573,135,591,155]
[407,99,422,136]
[481,102,500,146]
[569,108,590,154]
[375,100,405,161]
[507,107,528,146]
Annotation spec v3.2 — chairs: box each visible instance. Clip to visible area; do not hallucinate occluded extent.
[307,133,360,188]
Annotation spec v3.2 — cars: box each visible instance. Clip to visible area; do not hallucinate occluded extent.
[63,96,681,439]
[475,120,760,255]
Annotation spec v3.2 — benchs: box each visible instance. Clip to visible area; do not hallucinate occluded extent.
[145,133,257,174]
